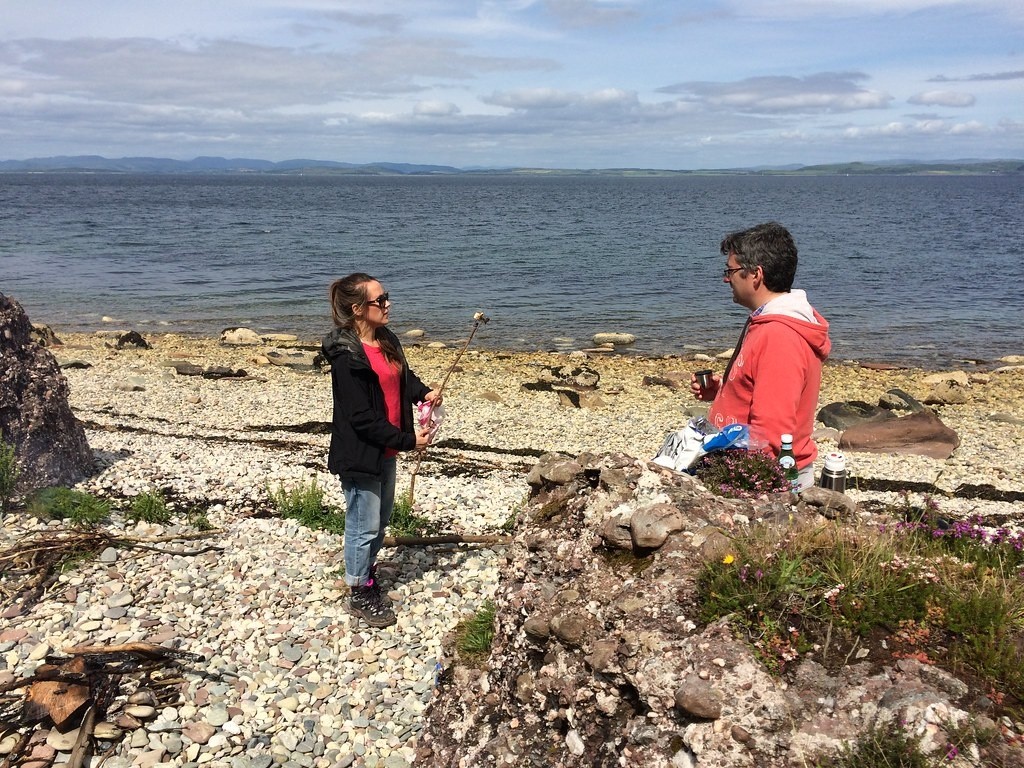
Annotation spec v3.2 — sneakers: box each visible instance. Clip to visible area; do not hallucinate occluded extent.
[369,565,392,608]
[350,579,397,627]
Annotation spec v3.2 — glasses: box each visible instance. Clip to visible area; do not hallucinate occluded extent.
[724,267,744,278]
[366,292,388,309]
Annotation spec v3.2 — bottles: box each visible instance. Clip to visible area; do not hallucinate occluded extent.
[776,434,797,496]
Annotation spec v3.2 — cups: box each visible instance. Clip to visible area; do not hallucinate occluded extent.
[695,370,713,390]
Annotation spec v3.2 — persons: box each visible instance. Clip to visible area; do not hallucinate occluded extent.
[322,273,442,628]
[689,222,831,492]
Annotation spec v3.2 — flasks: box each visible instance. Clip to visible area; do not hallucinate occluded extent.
[819,452,846,494]
